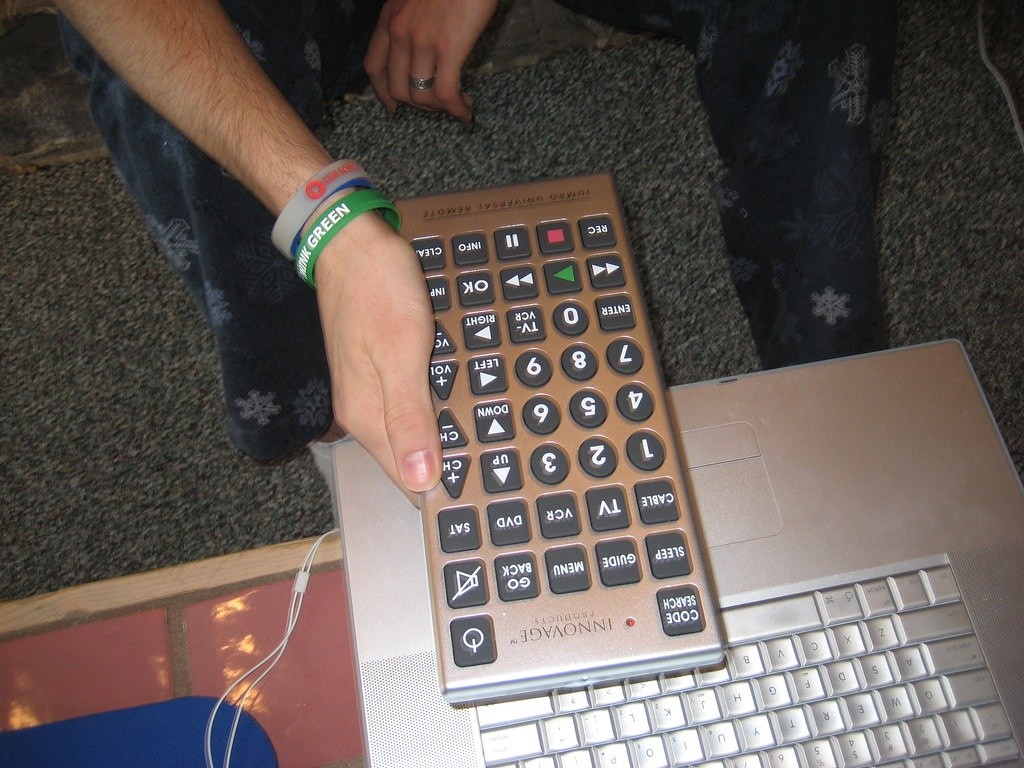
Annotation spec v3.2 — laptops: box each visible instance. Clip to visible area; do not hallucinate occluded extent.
[328,337,1024,768]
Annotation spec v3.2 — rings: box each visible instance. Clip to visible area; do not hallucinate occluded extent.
[408,74,434,91]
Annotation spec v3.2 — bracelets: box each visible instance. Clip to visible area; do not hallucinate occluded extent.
[271,158,402,288]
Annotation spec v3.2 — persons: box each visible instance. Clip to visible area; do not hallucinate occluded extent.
[55,0,900,529]
